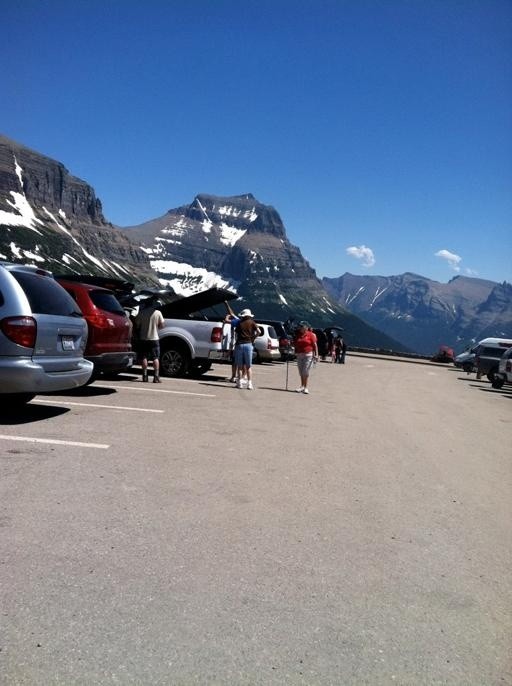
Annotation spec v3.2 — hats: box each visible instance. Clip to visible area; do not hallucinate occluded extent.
[238,309,255,318]
[297,320,312,327]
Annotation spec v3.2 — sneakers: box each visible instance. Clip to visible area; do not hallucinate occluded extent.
[296,386,309,393]
[143,375,148,382]
[231,377,254,389]
[153,375,161,383]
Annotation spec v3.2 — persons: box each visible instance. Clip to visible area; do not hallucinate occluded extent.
[234,308,262,390]
[336,334,346,364]
[288,320,319,395]
[135,297,164,382]
[330,342,338,364]
[224,298,248,385]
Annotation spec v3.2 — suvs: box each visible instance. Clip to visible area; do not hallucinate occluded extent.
[0,261,139,405]
[252,320,298,363]
[315,326,344,357]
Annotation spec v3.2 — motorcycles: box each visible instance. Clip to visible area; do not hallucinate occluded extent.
[492,347,512,388]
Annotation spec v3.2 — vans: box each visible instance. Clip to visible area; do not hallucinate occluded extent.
[453,338,512,381]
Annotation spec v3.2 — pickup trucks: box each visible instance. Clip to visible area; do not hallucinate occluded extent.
[118,287,239,377]
